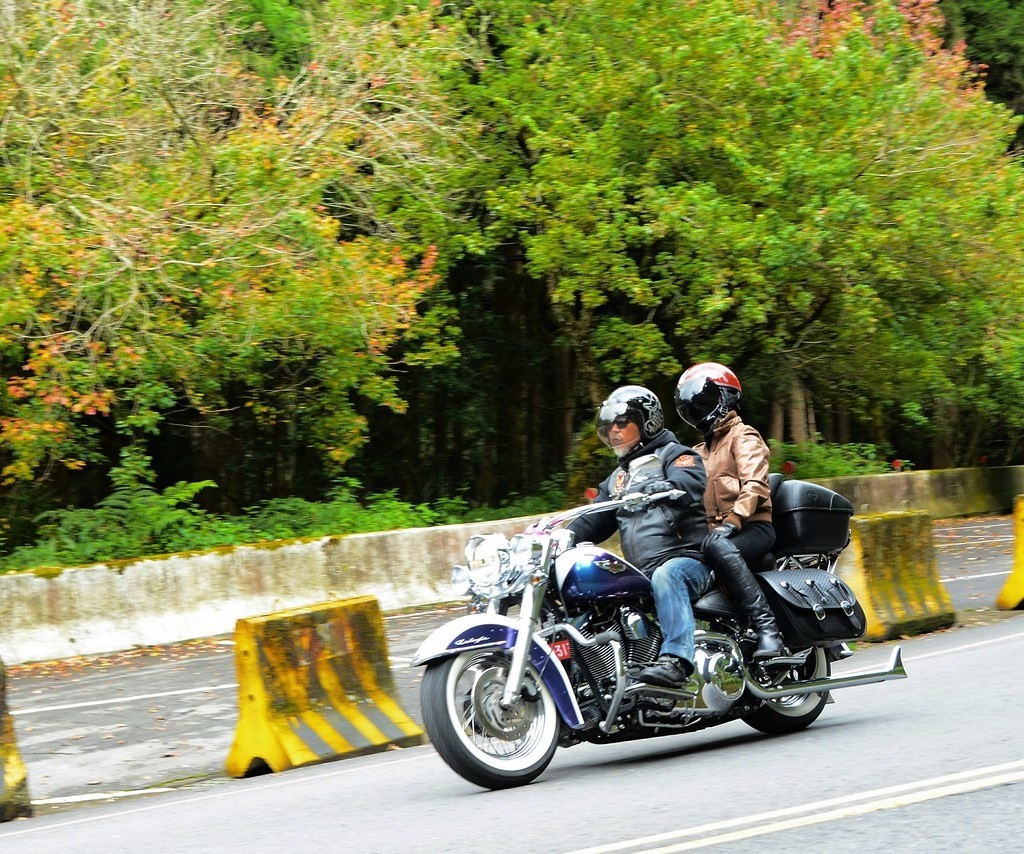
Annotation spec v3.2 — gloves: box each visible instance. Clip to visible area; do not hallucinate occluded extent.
[646,480,673,508]
[700,522,736,552]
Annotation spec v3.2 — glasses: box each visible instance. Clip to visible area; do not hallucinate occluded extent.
[603,416,635,432]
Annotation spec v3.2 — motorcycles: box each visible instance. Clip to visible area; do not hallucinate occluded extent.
[408,452,910,789]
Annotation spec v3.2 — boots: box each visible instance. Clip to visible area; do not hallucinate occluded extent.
[708,538,786,659]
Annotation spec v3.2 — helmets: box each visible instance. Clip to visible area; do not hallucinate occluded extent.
[677,361,742,394]
[597,385,664,439]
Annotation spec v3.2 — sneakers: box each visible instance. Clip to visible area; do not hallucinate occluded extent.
[639,657,687,687]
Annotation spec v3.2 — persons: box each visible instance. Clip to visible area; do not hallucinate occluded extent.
[564,385,716,690]
[674,362,785,661]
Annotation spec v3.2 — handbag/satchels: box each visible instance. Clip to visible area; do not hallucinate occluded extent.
[754,566,868,647]
